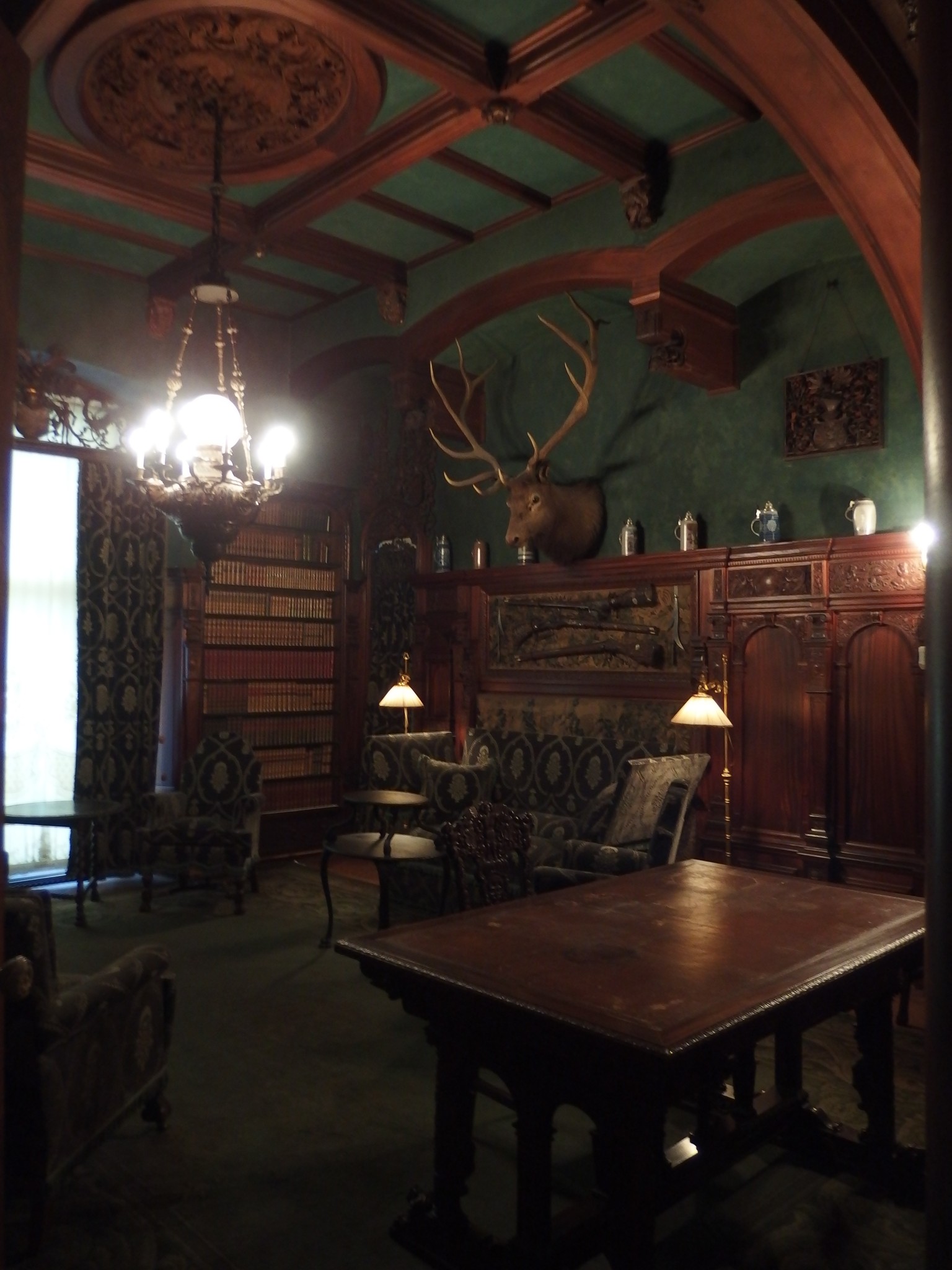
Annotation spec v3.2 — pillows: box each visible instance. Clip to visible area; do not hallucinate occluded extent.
[417,754,496,827]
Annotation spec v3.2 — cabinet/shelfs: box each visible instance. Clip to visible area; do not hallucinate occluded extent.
[154,498,368,859]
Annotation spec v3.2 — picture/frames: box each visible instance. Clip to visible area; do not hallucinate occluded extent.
[782,357,884,462]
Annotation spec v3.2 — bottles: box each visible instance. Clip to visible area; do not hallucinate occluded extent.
[435,533,451,573]
[674,511,699,551]
[472,537,488,569]
[751,500,780,545]
[619,517,638,556]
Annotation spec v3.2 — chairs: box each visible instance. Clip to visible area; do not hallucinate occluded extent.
[433,800,591,1105]
[141,736,262,914]
[0,848,177,1254]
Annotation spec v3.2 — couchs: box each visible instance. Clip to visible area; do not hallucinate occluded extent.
[364,729,711,883]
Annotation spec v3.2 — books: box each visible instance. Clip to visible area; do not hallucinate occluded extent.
[203,496,336,813]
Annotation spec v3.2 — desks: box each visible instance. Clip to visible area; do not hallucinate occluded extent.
[335,856,930,1270]
[3,794,150,927]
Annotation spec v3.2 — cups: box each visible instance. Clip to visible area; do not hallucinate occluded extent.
[845,499,877,536]
[517,546,536,566]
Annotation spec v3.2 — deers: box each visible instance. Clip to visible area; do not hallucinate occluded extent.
[429,291,605,561]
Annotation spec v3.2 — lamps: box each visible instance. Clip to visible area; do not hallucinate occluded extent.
[124,106,300,595]
[674,654,733,869]
[379,652,424,734]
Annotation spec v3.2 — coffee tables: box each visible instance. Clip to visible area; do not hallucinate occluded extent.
[318,789,438,946]
